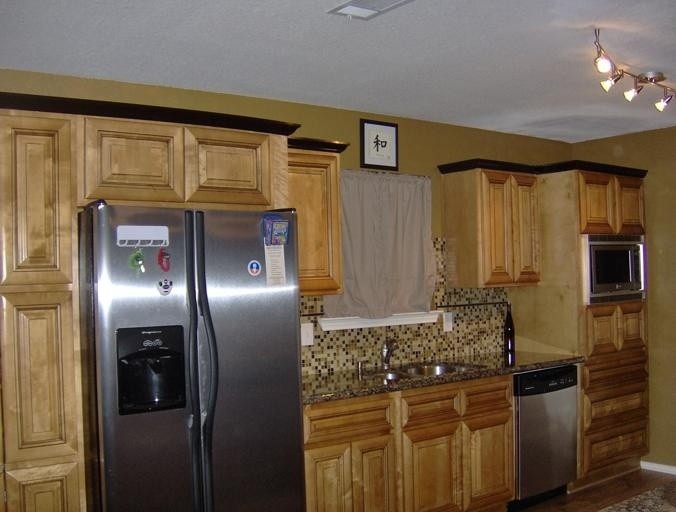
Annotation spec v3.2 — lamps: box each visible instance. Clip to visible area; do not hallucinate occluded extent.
[593,28,676,114]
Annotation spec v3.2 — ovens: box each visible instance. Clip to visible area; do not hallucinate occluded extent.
[515,364,578,503]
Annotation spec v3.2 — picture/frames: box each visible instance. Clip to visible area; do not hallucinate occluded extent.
[359,119,398,171]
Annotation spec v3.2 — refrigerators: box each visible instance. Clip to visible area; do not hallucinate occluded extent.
[76,197,309,511]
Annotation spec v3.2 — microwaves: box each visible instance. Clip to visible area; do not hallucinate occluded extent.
[584,231,646,302]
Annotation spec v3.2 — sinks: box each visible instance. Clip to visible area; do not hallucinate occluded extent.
[401,363,475,376]
[362,370,409,382]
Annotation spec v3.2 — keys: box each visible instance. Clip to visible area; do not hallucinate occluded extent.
[161,251,170,269]
[135,253,147,273]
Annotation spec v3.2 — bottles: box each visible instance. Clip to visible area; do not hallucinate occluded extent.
[503,302,517,367]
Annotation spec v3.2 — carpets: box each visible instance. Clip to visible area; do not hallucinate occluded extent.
[597,479,676,512]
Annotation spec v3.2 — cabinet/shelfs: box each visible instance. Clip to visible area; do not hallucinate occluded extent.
[289,149,340,293]
[579,352,649,487]
[302,388,400,511]
[512,299,649,354]
[440,169,541,286]
[400,373,516,512]
[82,113,290,209]
[2,109,89,509]
[541,169,649,235]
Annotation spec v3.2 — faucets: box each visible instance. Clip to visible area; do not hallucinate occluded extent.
[379,338,400,367]
